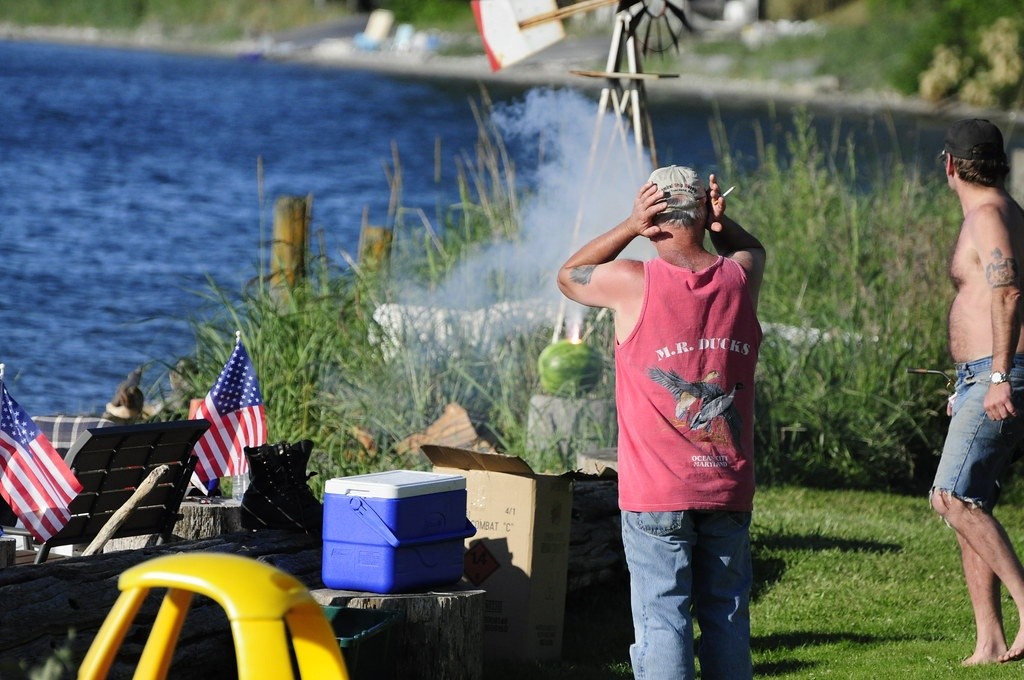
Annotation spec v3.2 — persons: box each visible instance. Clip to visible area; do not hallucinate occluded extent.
[929,118,1024,667]
[556,165,766,680]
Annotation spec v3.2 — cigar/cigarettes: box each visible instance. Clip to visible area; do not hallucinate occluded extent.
[714,185,737,207]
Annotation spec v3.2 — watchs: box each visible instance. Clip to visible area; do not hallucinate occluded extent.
[989,371,1012,385]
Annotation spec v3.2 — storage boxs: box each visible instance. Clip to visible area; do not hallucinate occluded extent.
[323,470,477,592]
[420,445,606,664]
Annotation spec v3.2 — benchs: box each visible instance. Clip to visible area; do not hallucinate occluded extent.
[0,419,213,567]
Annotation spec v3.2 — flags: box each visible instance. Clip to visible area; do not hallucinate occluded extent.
[0,375,83,545]
[190,337,267,485]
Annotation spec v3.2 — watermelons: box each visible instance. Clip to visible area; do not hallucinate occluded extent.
[539,341,602,397]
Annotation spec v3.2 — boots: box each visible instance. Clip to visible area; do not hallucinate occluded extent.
[239,441,323,533]
[274,438,324,523]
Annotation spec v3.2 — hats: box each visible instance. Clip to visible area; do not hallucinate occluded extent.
[647,163,707,203]
[938,118,1004,160]
